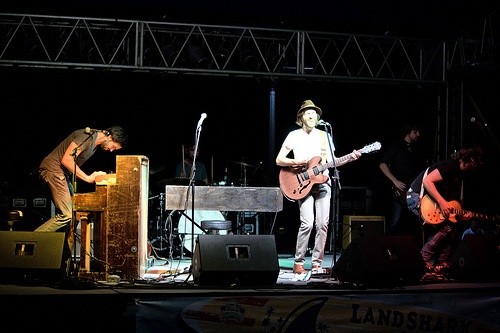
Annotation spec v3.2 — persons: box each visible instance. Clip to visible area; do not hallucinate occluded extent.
[175,144,209,185]
[405,145,486,281]
[379,125,436,243]
[276,100,361,274]
[33,127,127,255]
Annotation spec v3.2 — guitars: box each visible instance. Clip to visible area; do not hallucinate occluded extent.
[391,182,408,203]
[278,141,382,200]
[419,194,497,225]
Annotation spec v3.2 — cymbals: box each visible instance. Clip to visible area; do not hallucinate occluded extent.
[230,159,254,168]
[175,177,190,180]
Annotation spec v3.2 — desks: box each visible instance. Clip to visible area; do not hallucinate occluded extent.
[164,184,284,235]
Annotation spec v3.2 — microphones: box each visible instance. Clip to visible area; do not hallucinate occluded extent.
[318,120,329,126]
[85,127,107,133]
[196,113,207,128]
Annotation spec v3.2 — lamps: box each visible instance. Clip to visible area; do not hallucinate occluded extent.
[237,37,259,71]
[183,34,210,69]
[78,29,104,64]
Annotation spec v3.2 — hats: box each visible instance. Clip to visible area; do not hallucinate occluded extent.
[297,99,322,117]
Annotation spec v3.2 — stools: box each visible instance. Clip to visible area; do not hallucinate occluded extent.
[199,219,233,234]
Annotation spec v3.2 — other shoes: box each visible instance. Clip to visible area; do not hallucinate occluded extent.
[425,261,438,273]
[293,264,306,274]
[312,265,320,269]
[434,259,449,268]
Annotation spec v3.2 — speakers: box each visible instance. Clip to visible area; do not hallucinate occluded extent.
[0,230,74,282]
[332,238,421,288]
[337,187,372,238]
[343,216,385,249]
[191,234,280,287]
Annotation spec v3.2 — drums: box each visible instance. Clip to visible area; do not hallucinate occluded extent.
[164,209,229,257]
[208,180,235,188]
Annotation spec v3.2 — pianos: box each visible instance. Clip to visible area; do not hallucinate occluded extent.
[72,154,150,285]
